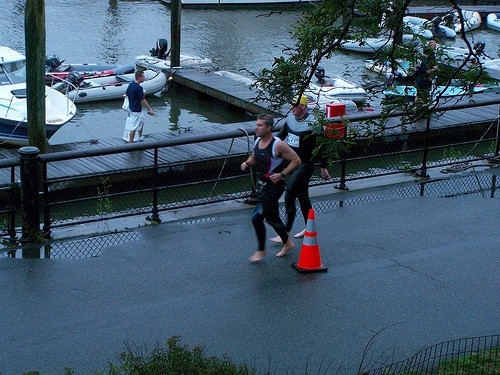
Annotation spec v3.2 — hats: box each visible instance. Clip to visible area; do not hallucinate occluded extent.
[291,94,307,104]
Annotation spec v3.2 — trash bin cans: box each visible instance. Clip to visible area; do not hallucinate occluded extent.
[325,101,346,138]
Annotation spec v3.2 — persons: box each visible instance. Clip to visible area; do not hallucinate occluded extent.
[126,70,154,144]
[240,113,301,263]
[428,40,439,103]
[412,44,438,111]
[269,94,331,243]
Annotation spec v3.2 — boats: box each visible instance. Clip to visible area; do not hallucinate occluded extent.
[296,92,358,116]
[419,43,485,70]
[482,58,500,80]
[364,57,441,78]
[215,68,253,87]
[46,61,135,87]
[442,10,482,34]
[310,75,368,105]
[401,15,458,39]
[382,77,491,98]
[51,68,167,103]
[0,45,77,147]
[487,13,500,32]
[134,51,213,72]
[323,31,421,54]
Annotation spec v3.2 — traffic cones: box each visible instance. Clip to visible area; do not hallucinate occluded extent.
[290,208,330,274]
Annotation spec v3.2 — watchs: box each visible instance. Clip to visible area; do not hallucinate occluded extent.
[280,172,286,180]
